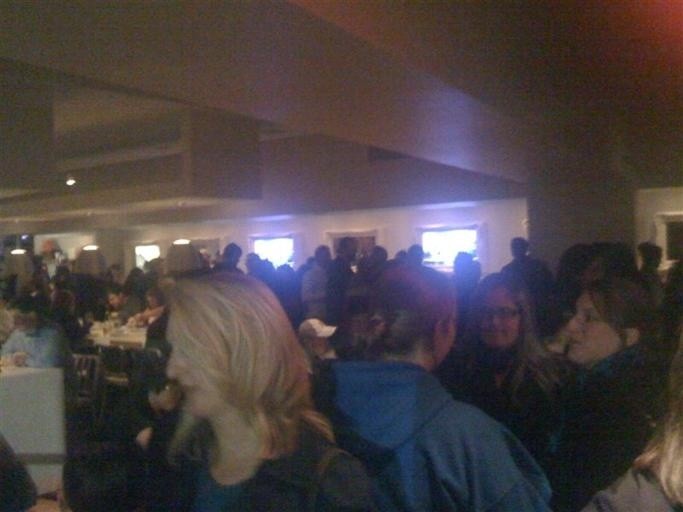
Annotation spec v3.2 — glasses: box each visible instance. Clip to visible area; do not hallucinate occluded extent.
[477,302,523,319]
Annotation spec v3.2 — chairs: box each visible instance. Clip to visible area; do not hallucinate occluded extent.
[66,319,148,440]
